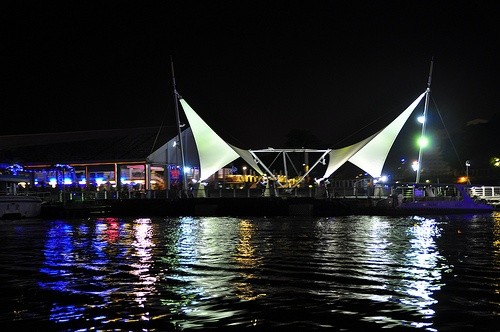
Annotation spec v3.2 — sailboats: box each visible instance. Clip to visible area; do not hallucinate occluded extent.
[367,56,494,213]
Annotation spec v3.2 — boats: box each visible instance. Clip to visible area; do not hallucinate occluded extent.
[0,174,46,220]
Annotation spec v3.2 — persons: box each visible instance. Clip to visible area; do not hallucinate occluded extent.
[21,174,462,202]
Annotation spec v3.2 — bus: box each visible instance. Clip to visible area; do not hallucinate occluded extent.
[25,192,51,202]
